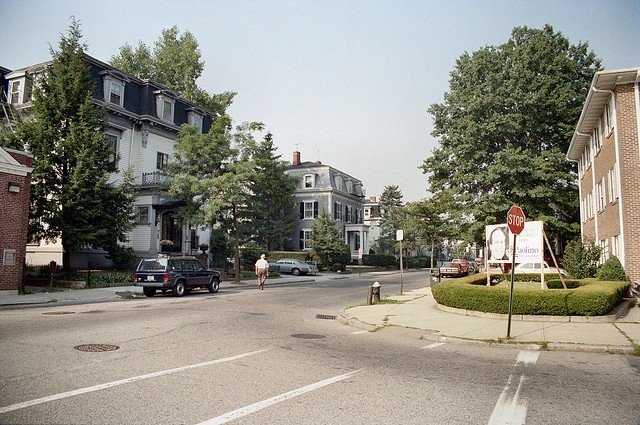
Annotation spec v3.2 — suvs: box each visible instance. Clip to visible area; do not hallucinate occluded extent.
[452,259,469,275]
[133,257,221,297]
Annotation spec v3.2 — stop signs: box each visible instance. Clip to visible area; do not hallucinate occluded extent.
[507,205,526,234]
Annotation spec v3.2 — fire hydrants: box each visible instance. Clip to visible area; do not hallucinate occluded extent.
[367,282,381,304]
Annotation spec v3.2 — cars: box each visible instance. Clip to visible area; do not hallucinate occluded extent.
[510,261,567,274]
[268,258,318,275]
[440,262,462,277]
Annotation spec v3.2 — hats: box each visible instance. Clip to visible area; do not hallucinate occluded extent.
[260,254,267,258]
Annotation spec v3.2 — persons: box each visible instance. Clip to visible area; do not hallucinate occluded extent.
[255,254,269,290]
[488,227,509,261]
